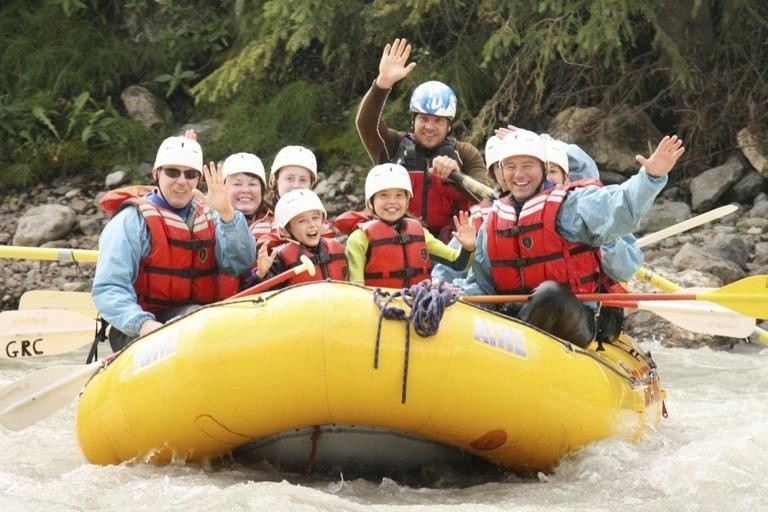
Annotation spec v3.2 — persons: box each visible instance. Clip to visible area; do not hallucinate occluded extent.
[246,144,348,255]
[345,162,476,292]
[432,122,685,346]
[354,36,493,244]
[91,134,256,352]
[179,128,273,245]
[244,189,349,292]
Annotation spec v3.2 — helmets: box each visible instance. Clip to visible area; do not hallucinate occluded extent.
[271,146,317,183]
[154,137,203,175]
[365,163,413,210]
[223,152,266,188]
[410,81,456,117]
[275,189,327,237]
[485,130,569,173]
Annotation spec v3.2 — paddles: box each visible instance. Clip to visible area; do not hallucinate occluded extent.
[598,287,757,339]
[457,274,768,320]
[637,204,739,249]
[1,254,316,432]
[20,290,105,322]
[2,310,109,356]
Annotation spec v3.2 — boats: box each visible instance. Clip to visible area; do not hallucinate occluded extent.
[74,278,668,479]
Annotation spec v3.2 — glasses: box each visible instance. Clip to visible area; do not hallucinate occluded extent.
[165,168,198,178]
